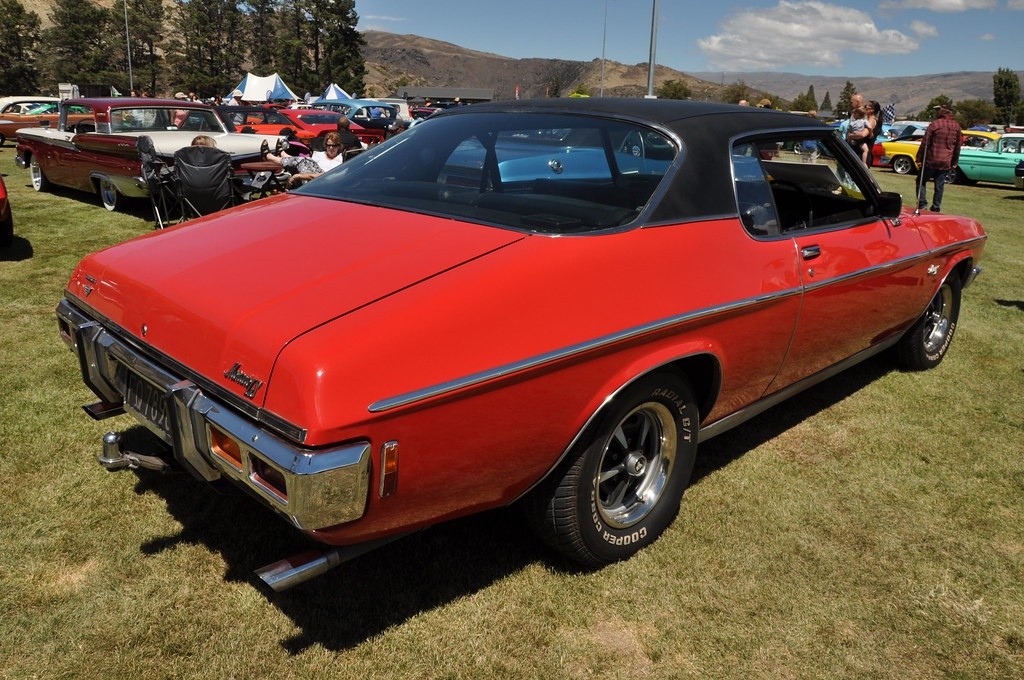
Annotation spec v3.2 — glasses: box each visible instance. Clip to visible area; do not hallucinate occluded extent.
[327,144,337,147]
[864,105,873,110]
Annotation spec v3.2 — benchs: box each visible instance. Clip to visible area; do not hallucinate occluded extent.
[385,181,637,229]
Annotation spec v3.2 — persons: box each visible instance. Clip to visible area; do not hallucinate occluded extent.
[756,99,779,160]
[370,107,389,118]
[802,110,817,162]
[210,94,222,106]
[227,89,244,123]
[130,89,137,96]
[739,100,749,106]
[192,135,218,147]
[261,116,362,184]
[916,102,962,212]
[832,94,884,194]
[175,91,203,103]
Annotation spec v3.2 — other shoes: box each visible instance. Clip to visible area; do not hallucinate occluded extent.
[275,138,285,157]
[920,205,929,210]
[261,139,271,162]
[931,207,940,212]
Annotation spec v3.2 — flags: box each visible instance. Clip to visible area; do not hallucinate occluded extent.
[112,86,122,97]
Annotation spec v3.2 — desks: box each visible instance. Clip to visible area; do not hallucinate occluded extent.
[241,161,283,198]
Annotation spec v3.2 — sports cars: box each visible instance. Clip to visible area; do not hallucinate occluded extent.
[47,96,989,590]
[14,96,290,212]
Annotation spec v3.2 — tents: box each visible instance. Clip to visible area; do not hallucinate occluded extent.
[225,72,303,101]
[315,83,353,101]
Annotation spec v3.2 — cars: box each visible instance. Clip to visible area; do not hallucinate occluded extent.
[866,121,1001,174]
[215,106,317,152]
[0,96,142,146]
[951,133,1024,190]
[310,99,443,140]
[432,121,775,207]
[263,104,384,148]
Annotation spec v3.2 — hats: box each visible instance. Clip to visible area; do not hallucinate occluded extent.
[233,90,243,97]
[755,99,772,107]
[933,102,954,110]
[175,92,188,98]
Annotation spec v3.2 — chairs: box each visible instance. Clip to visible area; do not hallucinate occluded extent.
[170,145,236,223]
[134,135,175,230]
